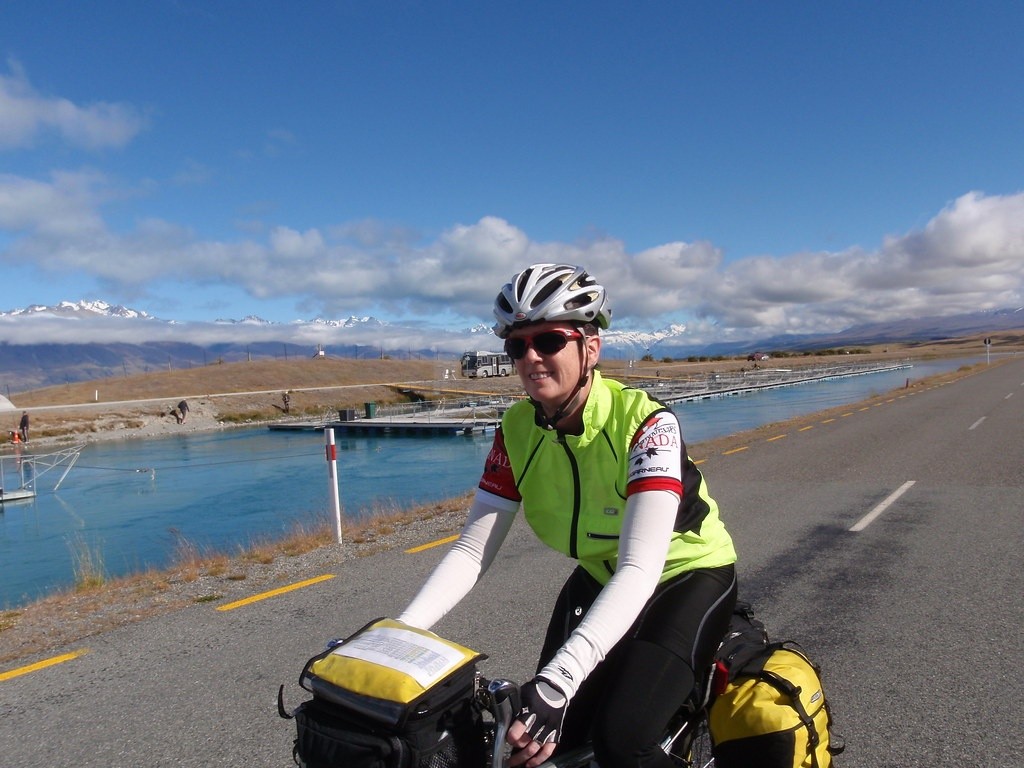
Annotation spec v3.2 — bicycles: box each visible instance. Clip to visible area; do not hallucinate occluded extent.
[325,608,783,768]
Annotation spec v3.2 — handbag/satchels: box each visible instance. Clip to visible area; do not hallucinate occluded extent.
[289,615,482,768]
[707,640,846,768]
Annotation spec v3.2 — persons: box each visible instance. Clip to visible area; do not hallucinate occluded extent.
[178,398,190,424]
[393,262,743,768]
[20,411,30,444]
[655,371,660,377]
[282,391,292,413]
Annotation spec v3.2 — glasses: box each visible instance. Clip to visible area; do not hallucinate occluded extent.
[504,329,592,359]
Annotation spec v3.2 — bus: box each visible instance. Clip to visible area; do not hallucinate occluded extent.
[460,351,513,379]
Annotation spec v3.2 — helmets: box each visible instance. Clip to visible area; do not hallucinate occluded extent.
[492,263,611,339]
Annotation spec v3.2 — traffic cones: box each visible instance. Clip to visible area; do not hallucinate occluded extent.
[10,430,19,444]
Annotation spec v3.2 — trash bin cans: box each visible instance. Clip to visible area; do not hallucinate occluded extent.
[339,410,356,422]
[365,402,377,419]
